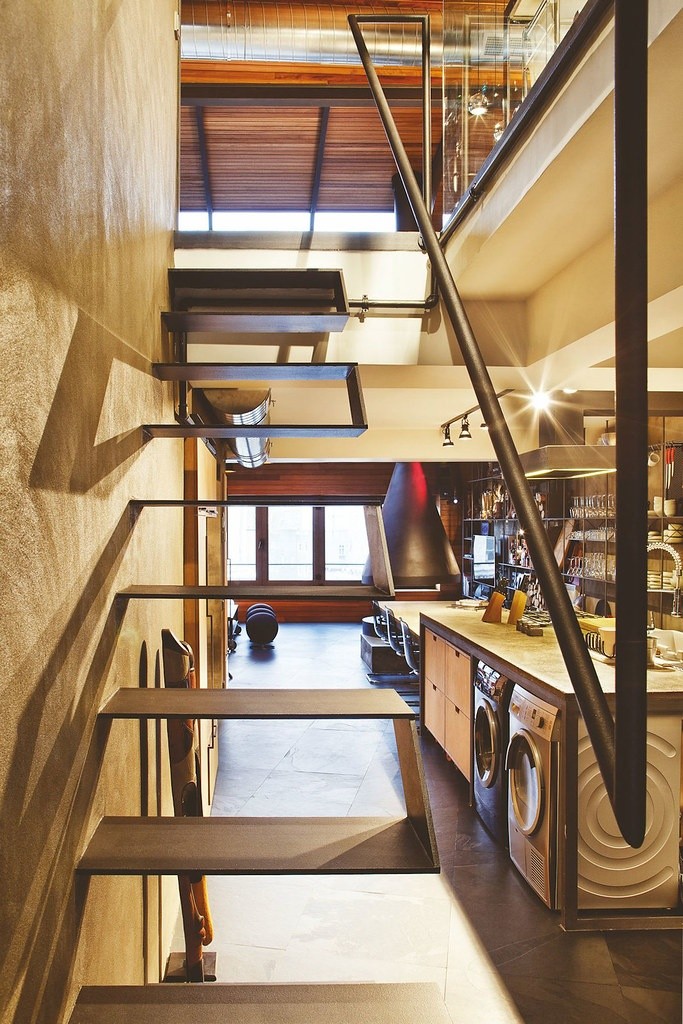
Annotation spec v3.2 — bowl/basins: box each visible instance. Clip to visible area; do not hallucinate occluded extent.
[653,496,675,517]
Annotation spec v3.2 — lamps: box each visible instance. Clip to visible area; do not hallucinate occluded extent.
[459,414,472,441]
[442,423,455,448]
[494,120,504,141]
[468,0,489,116]
[480,423,488,430]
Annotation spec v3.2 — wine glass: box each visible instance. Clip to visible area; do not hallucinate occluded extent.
[570,495,616,517]
[567,551,616,579]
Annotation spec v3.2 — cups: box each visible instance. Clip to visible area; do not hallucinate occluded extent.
[598,627,617,657]
[664,522,683,543]
[566,527,616,539]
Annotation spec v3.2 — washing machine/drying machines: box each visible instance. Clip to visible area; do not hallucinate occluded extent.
[508,683,681,912]
[473,659,507,844]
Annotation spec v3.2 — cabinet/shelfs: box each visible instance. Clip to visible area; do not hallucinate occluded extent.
[419,417,683,933]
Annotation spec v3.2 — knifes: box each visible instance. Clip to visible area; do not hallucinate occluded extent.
[486,576,509,597]
[518,574,530,593]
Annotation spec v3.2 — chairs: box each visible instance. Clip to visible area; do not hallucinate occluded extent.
[371,599,420,730]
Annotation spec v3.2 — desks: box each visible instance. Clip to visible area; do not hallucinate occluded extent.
[378,601,457,644]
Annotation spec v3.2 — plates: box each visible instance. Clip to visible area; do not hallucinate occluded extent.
[647,570,674,589]
[647,531,662,544]
